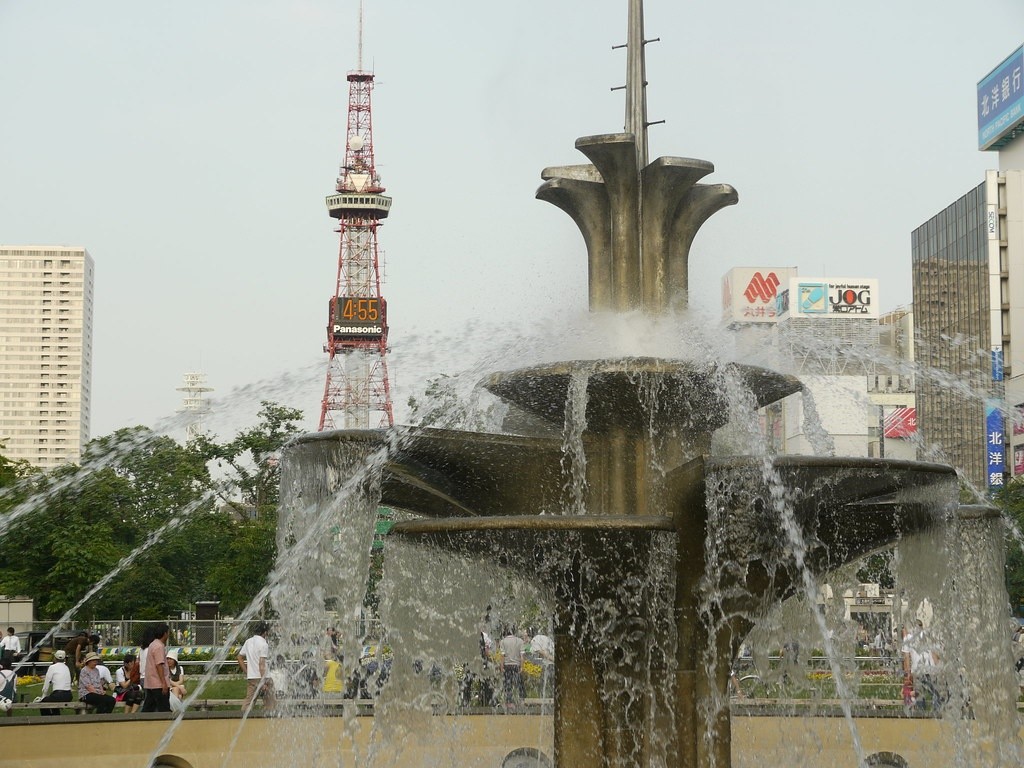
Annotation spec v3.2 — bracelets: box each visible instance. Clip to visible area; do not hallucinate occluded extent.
[737,691,740,693]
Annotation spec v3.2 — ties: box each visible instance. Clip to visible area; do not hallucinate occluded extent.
[10,637,11,651]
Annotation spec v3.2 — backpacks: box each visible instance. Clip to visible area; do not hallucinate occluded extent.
[0,670,17,700]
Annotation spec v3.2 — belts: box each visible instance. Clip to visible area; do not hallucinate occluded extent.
[55,690,72,692]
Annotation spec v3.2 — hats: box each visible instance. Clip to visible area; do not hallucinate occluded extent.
[85,652,101,664]
[166,650,178,664]
[54,649,66,659]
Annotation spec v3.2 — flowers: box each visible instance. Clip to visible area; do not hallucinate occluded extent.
[363,644,543,685]
[17,674,45,685]
[806,670,899,681]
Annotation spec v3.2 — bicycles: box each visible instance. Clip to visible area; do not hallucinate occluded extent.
[726,655,770,700]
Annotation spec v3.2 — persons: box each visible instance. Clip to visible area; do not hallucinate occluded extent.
[138,626,156,693]
[63,631,114,684]
[39,650,73,716]
[236,620,278,718]
[730,669,744,699]
[166,650,188,701]
[78,651,116,714]
[140,624,170,712]
[115,654,142,713]
[0,626,22,660]
[271,621,555,701]
[0,656,18,716]
[892,616,947,708]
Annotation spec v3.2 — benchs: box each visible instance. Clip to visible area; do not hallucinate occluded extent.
[8,698,1024,716]
